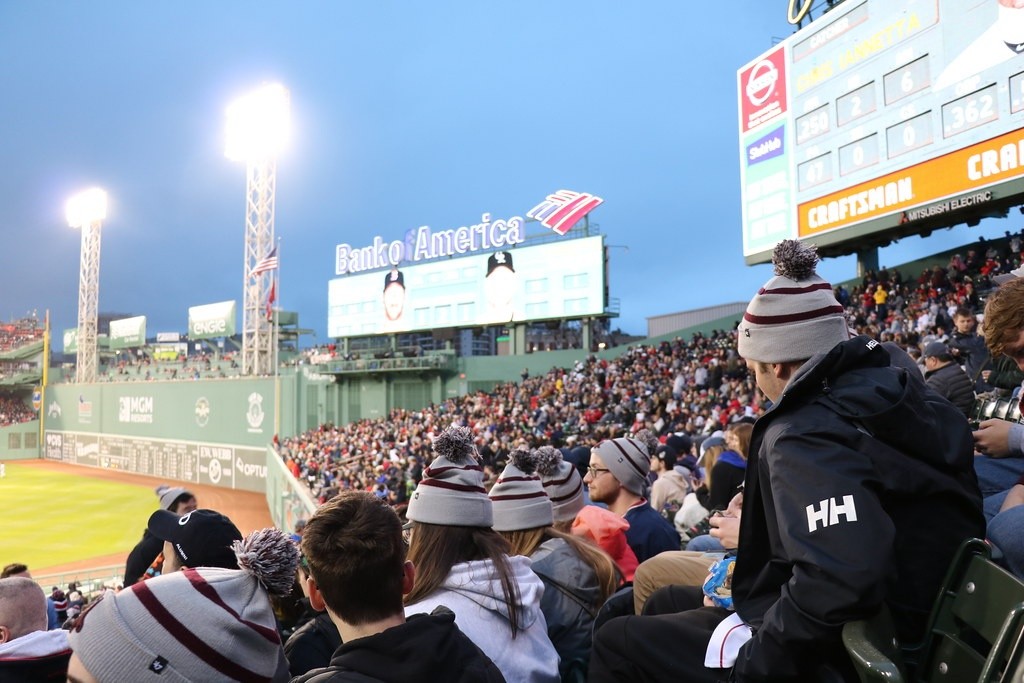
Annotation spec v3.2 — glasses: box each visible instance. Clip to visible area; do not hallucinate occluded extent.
[587,466,609,478]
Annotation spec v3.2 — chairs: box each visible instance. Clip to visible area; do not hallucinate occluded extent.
[838,536,1024,683]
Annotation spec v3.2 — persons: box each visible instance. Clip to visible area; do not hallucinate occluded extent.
[289,493,507,683]
[0,231,1024,683]
[477,251,525,324]
[66,527,301,683]
[378,270,411,331]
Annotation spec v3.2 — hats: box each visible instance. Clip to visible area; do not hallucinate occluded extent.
[51,590,81,620]
[917,342,947,365]
[737,241,849,363]
[154,484,190,510]
[69,527,301,683]
[406,426,493,526]
[534,446,585,521]
[487,450,554,531]
[147,510,243,569]
[590,429,656,496]
[695,437,726,467]
